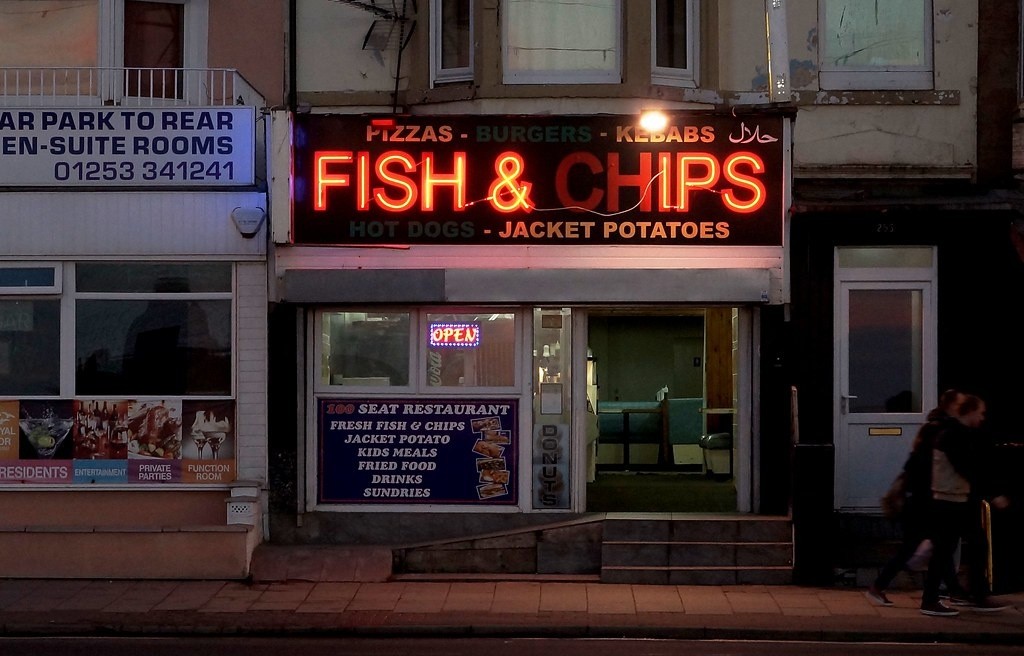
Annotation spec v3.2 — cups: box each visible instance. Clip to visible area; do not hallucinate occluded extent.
[19,419,73,460]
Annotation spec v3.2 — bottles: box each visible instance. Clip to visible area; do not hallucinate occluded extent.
[76,401,119,441]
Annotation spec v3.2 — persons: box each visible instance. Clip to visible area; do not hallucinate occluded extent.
[864,390,1008,615]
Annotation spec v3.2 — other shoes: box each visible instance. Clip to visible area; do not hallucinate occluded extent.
[865,589,894,606]
[921,600,959,616]
[971,600,1011,612]
[949,598,971,607]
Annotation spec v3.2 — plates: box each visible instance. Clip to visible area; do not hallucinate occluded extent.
[128,440,182,460]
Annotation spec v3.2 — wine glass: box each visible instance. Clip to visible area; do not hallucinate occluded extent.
[191,435,207,460]
[200,432,227,461]
